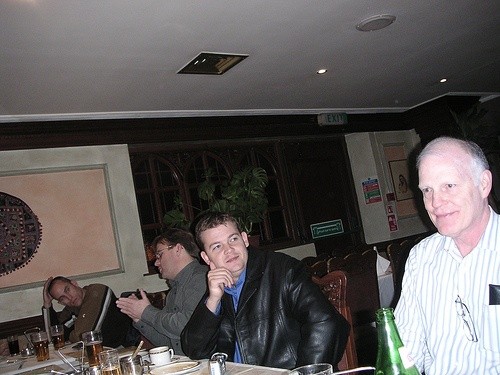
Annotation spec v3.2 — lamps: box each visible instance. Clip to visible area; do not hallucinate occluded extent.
[356,13,397,32]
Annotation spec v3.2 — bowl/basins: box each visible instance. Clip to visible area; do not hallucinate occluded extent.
[21,347,34,356]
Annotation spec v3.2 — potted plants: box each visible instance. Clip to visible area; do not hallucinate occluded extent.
[163,165,271,248]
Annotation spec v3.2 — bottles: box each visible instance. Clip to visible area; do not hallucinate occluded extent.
[373,306,421,375]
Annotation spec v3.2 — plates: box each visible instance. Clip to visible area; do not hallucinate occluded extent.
[143,356,180,367]
[119,350,149,358]
[148,360,203,375]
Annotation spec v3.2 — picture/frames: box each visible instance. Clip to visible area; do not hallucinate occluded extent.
[0,163,125,295]
[383,142,420,220]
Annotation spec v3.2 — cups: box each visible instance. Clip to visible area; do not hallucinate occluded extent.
[82,329,104,375]
[119,354,144,375]
[30,332,50,362]
[7,334,21,357]
[286,362,333,375]
[98,349,122,375]
[149,346,174,364]
[49,324,66,351]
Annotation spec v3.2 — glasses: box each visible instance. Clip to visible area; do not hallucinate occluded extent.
[155,244,184,258]
[455,297,478,342]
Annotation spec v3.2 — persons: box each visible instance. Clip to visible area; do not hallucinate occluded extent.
[391,136,500,375]
[41,276,141,348]
[116,229,211,356]
[180,211,350,375]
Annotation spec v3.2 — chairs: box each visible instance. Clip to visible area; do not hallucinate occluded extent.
[301,236,424,375]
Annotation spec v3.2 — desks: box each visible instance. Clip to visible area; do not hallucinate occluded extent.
[0,341,292,375]
